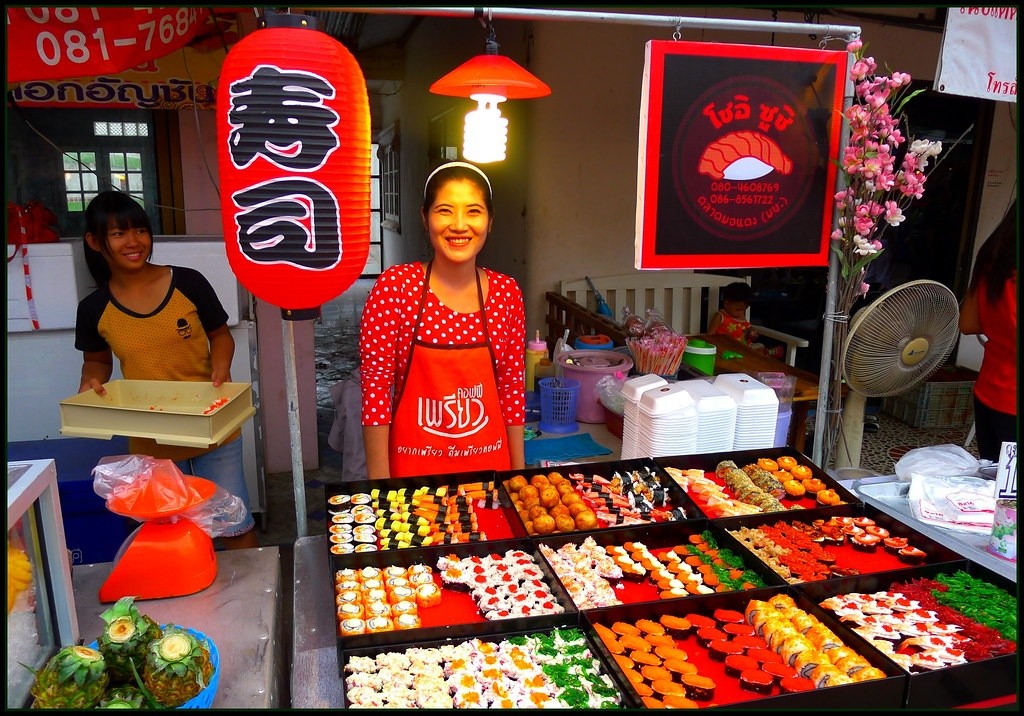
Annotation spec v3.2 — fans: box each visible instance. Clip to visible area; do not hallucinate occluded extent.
[830,278,959,469]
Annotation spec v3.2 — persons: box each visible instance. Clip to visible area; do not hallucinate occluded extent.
[708,282,784,359]
[958,200,1017,463]
[75,191,259,550]
[358,159,526,479]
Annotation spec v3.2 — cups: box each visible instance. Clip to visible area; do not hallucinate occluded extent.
[773,408,792,448]
[634,370,677,384]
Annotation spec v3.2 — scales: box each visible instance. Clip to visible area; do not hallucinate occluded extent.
[99,475,219,607]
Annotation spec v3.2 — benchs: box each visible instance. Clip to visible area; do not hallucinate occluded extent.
[559,272,810,368]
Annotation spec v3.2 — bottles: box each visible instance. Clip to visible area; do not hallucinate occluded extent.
[534,352,554,394]
[526,330,549,391]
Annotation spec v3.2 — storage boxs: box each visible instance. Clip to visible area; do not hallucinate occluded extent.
[57,380,256,450]
[323,444,1019,711]
[6,235,251,333]
[880,364,980,429]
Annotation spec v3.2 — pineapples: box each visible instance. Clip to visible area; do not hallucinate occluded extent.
[17,593,215,709]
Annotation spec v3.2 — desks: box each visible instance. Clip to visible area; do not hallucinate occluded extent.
[667,333,852,456]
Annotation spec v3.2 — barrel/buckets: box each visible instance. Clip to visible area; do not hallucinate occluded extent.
[557,350,633,424]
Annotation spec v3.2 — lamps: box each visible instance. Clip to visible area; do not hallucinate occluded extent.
[429,23,554,164]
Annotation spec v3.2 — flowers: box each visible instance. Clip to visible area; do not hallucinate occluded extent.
[822,37,942,474]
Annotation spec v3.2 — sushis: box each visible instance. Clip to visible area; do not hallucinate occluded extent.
[329,482,499,554]
[590,594,886,709]
[725,515,926,584]
[568,466,687,527]
[814,591,993,675]
[714,456,849,514]
[335,549,624,708]
[539,530,766,600]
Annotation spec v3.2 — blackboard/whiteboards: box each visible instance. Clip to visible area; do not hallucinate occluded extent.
[863,80,995,296]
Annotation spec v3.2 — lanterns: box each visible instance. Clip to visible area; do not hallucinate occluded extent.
[215,12,371,321]
